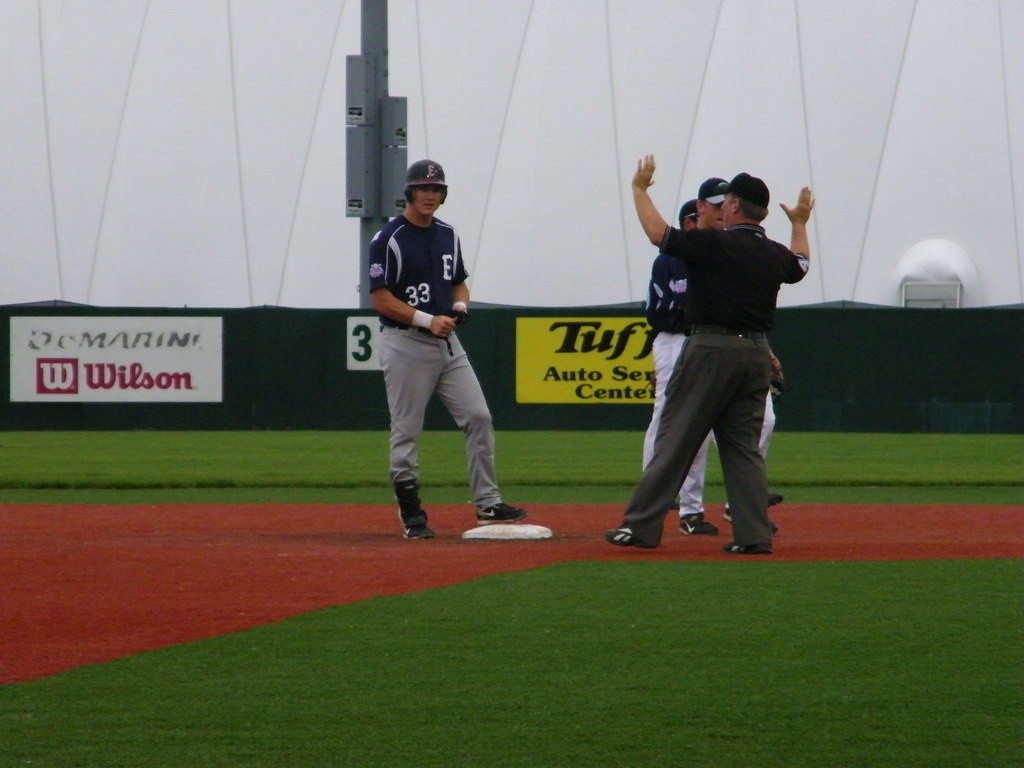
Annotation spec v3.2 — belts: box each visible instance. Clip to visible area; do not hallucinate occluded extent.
[692,325,764,339]
[379,325,435,338]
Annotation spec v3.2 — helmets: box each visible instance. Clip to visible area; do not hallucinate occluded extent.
[404,159,448,205]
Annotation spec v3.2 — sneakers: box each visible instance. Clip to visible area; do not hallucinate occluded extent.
[403,526,435,539]
[678,519,718,535]
[605,530,655,547]
[722,541,772,553]
[768,493,784,505]
[724,509,779,534]
[477,502,527,525]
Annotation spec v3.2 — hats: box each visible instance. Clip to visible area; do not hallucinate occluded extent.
[678,199,697,217]
[722,172,770,208]
[698,177,730,204]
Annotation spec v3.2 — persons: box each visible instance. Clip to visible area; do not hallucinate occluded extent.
[368,159,531,539]
[602,153,815,555]
[630,176,785,536]
